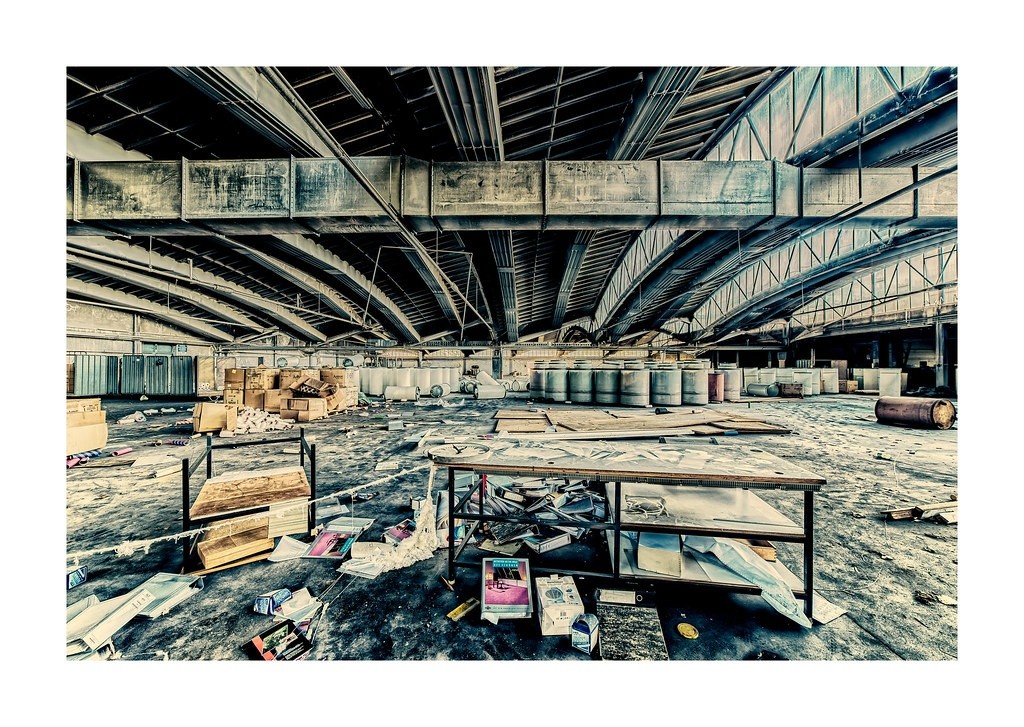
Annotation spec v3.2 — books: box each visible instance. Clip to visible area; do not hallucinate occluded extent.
[384,518,418,545]
[439,471,610,555]
[301,516,375,559]
[636,531,685,578]
[736,538,776,562]
[481,557,533,620]
[248,586,330,661]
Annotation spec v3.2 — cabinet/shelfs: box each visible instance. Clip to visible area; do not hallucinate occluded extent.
[432,435,827,624]
[182,427,317,573]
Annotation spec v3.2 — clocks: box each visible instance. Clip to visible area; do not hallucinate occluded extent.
[428,443,492,464]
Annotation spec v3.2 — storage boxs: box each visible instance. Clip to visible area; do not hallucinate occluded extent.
[192,363,362,432]
[66,399,107,456]
[839,380,859,394]
[780,382,804,398]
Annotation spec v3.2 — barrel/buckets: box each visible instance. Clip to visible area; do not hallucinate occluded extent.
[623,370,650,406]
[652,369,682,406]
[533,358,737,370]
[793,372,813,395]
[710,374,725,403]
[544,368,568,401]
[726,370,740,400]
[796,366,957,398]
[761,368,776,386]
[530,368,545,398]
[744,369,759,389]
[685,371,709,405]
[570,370,592,402]
[778,368,794,385]
[595,369,621,403]
